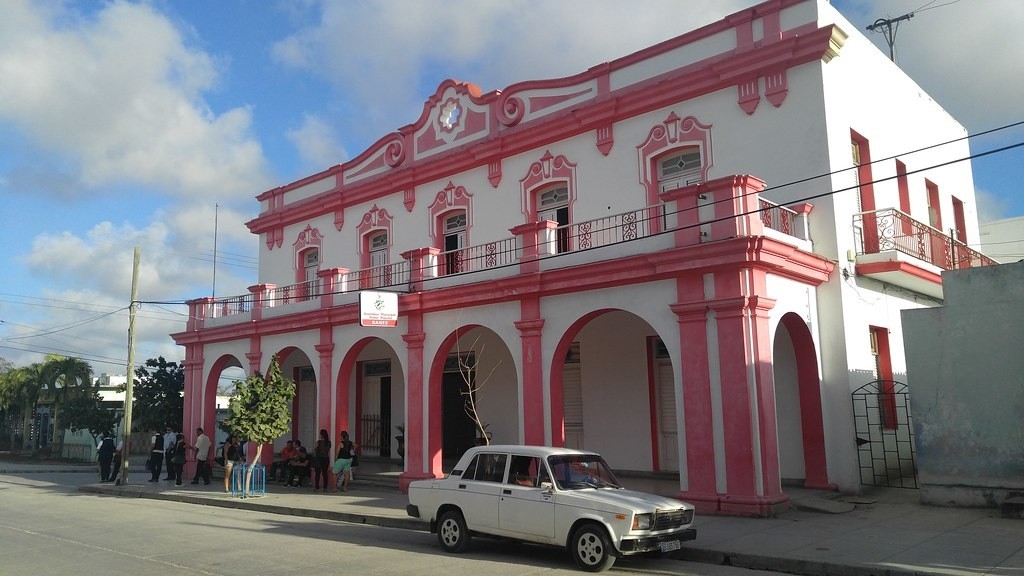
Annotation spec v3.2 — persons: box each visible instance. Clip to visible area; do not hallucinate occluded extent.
[164,424,176,481]
[224,434,244,494]
[332,431,357,493]
[108,435,123,482]
[172,435,197,487]
[96,430,116,483]
[149,426,164,482]
[190,428,212,485]
[313,429,331,492]
[267,439,314,488]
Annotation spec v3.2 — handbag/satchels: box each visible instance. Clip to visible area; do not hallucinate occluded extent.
[145,456,152,470]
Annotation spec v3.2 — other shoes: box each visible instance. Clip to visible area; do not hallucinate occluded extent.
[99,480,104,483]
[225,490,230,493]
[328,489,337,492]
[148,478,158,482]
[106,481,109,482]
[268,477,275,481]
[279,478,284,482]
[175,483,186,488]
[283,483,292,487]
[341,488,346,491]
[293,481,302,487]
[204,481,210,485]
[163,477,171,480]
[191,481,198,484]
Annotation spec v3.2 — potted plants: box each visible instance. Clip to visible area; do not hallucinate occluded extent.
[472,421,493,448]
[394,423,405,466]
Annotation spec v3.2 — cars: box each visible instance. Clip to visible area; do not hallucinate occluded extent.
[406,445,697,573]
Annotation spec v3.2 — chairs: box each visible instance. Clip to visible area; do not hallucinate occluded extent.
[511,470,534,486]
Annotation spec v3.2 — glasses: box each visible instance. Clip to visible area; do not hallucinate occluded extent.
[287,445,291,446]
[165,429,171,431]
